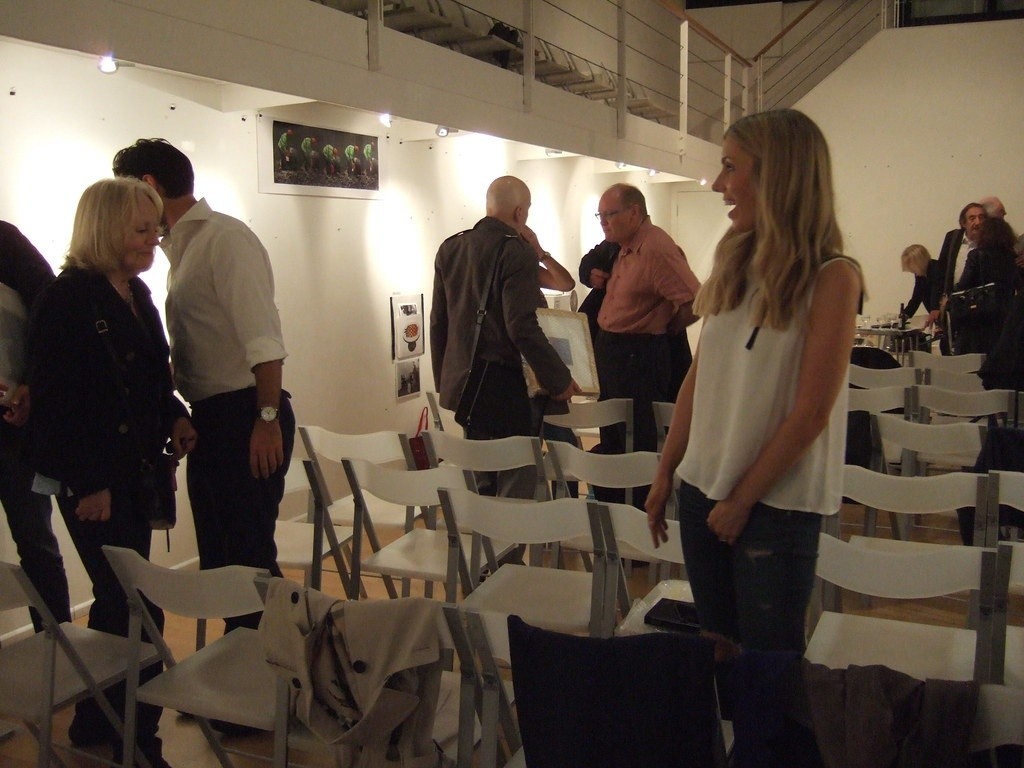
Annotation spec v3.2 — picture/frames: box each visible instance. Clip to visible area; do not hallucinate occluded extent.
[520,307,601,398]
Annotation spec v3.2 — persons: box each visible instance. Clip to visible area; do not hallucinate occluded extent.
[577,183,702,566]
[24,179,197,768]
[900,195,1024,392]
[645,109,863,768]
[429,176,582,590]
[112,138,296,739]
[0,220,73,635]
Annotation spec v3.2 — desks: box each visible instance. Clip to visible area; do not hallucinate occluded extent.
[856,327,925,366]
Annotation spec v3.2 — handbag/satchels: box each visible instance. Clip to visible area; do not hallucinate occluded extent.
[409,407,430,470]
[146,457,176,530]
[455,359,546,435]
[949,253,1000,326]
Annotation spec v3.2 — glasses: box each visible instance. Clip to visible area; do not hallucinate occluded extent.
[596,207,632,219]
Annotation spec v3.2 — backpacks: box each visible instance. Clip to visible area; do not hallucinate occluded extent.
[959,389,1024,546]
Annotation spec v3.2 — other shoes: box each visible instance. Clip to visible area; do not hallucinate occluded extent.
[111,737,172,768]
[69,713,128,744]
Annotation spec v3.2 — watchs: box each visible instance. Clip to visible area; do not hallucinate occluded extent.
[540,251,551,261]
[258,407,280,422]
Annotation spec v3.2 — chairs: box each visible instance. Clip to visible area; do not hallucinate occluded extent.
[0,350,1024,768]
[313,0,677,124]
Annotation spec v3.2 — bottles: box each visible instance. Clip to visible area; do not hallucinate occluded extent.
[898,303,905,330]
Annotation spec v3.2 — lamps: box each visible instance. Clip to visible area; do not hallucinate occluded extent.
[98,56,136,73]
[436,126,459,136]
